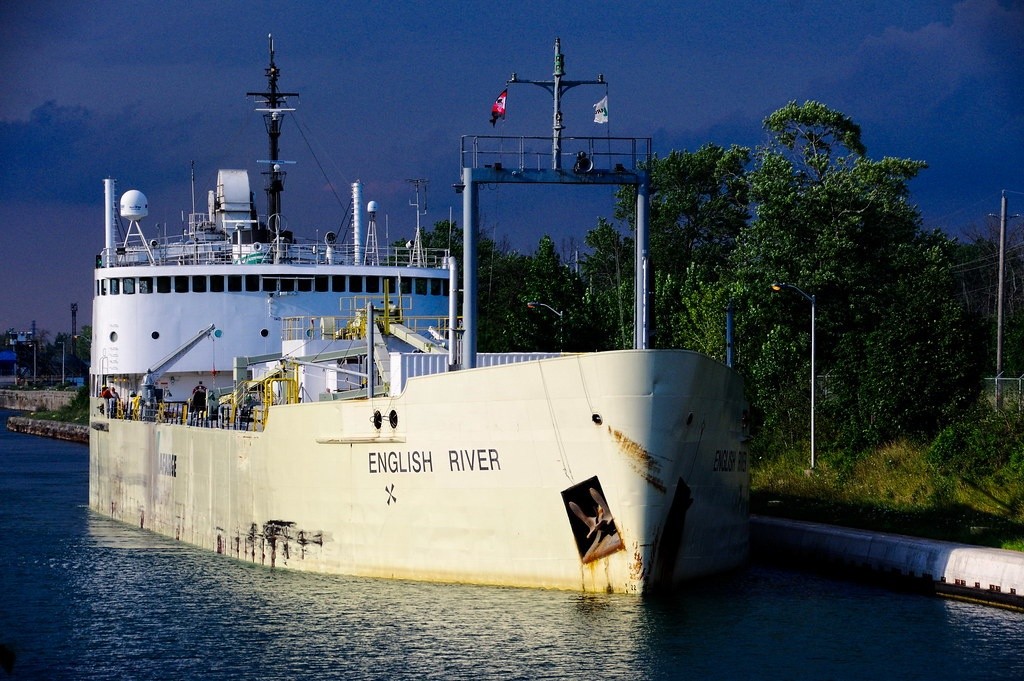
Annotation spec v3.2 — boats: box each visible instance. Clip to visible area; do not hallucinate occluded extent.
[86,34,754,596]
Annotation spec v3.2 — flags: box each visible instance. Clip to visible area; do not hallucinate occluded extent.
[591,94,608,123]
[489,89,507,127]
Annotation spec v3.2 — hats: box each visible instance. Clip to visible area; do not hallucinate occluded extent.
[101,384,107,388]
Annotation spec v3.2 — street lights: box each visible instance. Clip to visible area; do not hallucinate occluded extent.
[527,302,564,352]
[771,281,815,470]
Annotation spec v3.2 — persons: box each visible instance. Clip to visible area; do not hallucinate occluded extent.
[191,380,207,418]
[101,385,113,397]
[108,387,120,419]
